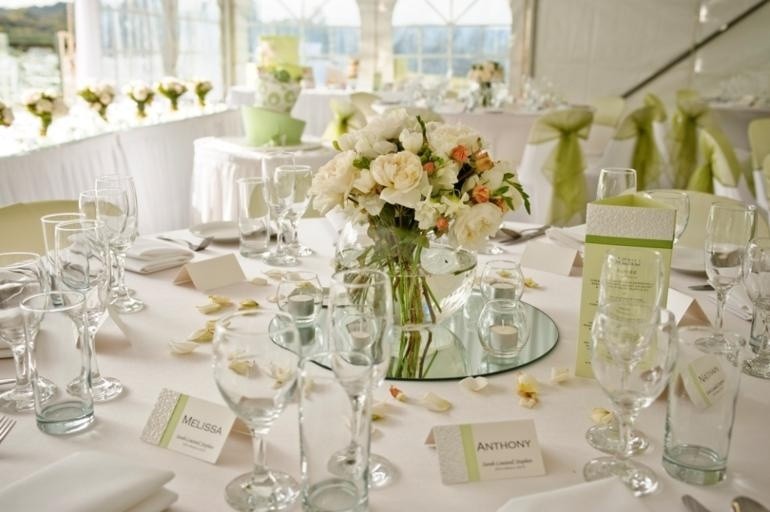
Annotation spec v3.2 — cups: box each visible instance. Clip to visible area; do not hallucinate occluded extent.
[297,350,374,511]
[405,78,563,112]
[597,167,691,243]
[237,174,271,260]
[660,324,744,486]
[19,290,97,438]
[276,260,531,361]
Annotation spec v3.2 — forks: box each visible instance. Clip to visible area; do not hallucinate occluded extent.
[160,234,214,253]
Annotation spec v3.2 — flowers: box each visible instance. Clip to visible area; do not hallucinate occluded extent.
[157,77,214,108]
[469,59,506,99]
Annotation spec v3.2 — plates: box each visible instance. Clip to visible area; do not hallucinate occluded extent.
[190,220,265,244]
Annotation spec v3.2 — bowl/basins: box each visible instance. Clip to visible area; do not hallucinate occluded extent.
[337,244,474,327]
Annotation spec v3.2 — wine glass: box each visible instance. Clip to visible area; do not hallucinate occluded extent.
[0,174,145,413]
[703,199,768,380]
[263,150,315,270]
[585,246,678,499]
[325,267,396,486]
[212,308,302,512]
